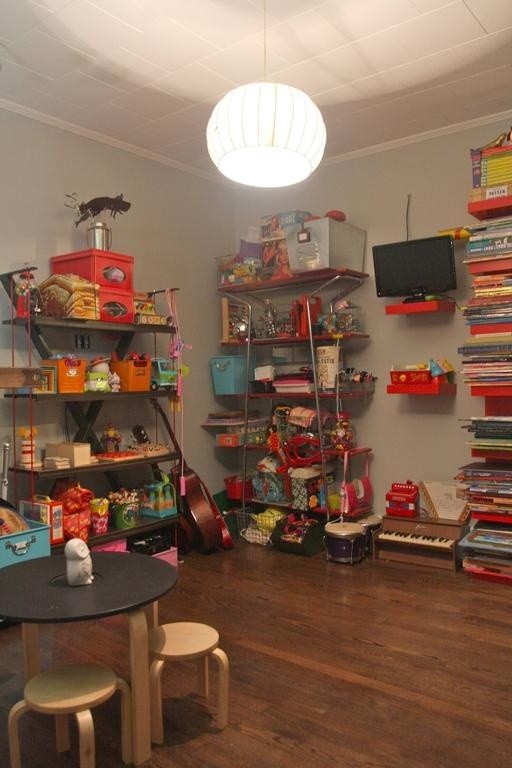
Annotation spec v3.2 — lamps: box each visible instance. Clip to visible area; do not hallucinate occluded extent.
[206,0,327,189]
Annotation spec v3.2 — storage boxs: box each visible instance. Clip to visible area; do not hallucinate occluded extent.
[210,356,262,395]
[0,519,51,570]
[40,359,85,394]
[19,493,65,545]
[50,248,134,290]
[89,525,178,567]
[284,217,367,274]
[109,361,151,392]
[45,441,91,468]
[99,286,135,324]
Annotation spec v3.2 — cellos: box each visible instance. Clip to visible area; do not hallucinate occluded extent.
[149,397,216,555]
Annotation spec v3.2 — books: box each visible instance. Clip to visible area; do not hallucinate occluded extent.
[457,126,512,587]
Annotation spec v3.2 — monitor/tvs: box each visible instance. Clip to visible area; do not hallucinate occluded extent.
[372,234,457,303]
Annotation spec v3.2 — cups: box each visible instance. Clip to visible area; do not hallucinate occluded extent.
[90,498,109,534]
[88,221,113,251]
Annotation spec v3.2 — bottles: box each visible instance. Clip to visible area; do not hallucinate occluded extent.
[14,274,41,316]
[262,299,278,336]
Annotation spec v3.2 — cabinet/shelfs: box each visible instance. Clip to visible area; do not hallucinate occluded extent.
[467,194,512,587]
[217,268,373,518]
[0,265,179,551]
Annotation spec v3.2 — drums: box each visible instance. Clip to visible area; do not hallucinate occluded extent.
[351,516,382,555]
[322,521,366,564]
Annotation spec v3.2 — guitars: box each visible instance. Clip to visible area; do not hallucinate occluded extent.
[188,468,233,550]
[132,425,195,554]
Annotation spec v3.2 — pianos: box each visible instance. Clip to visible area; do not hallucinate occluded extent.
[372,514,470,572]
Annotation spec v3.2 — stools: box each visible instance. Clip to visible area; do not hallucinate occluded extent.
[148,622,229,744]
[8,663,132,767]
